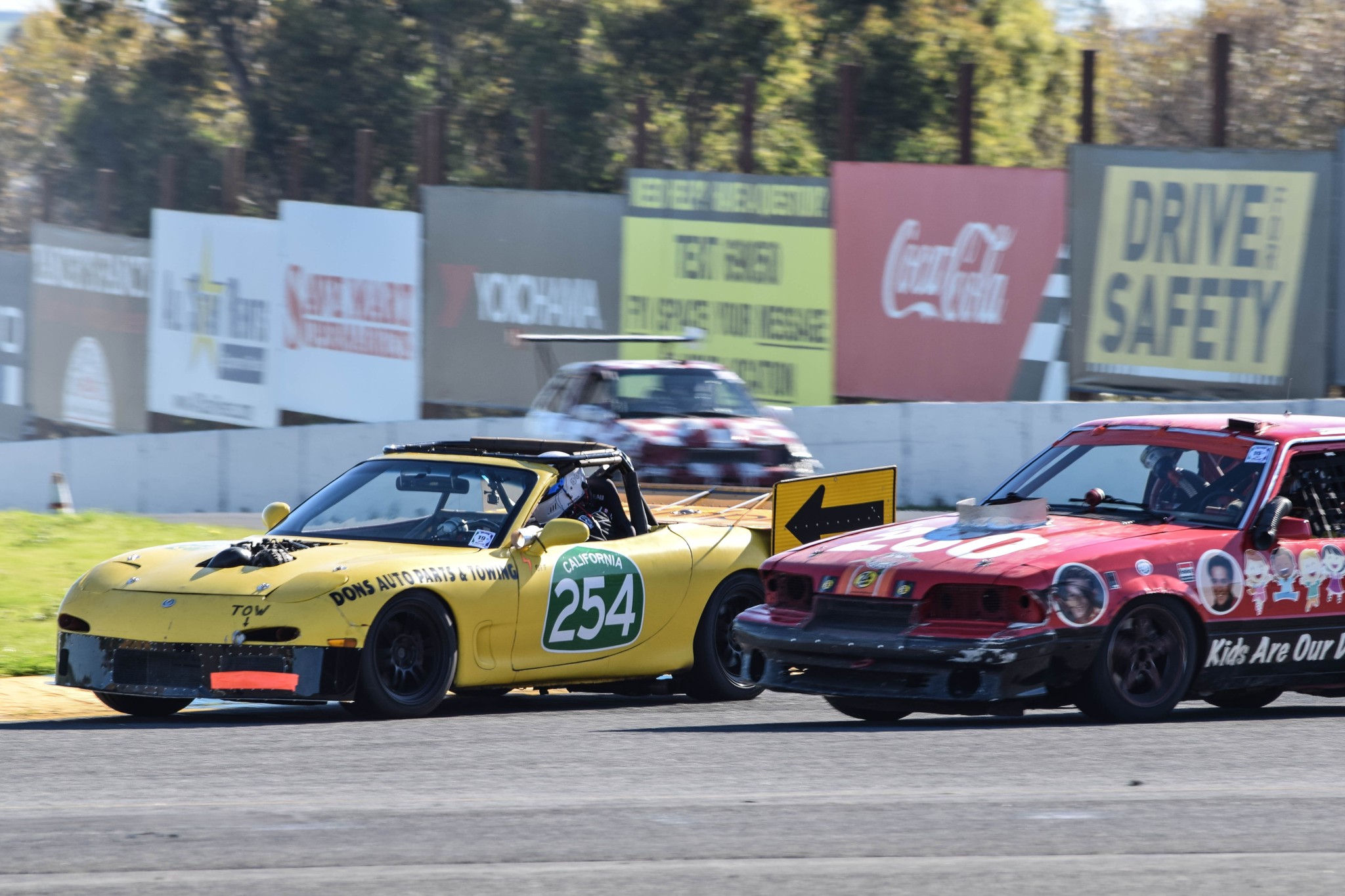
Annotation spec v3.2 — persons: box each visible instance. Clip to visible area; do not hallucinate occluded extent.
[437,450,617,548]
[659,372,721,412]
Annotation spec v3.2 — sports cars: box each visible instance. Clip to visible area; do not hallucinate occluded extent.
[733,409,1345,724]
[515,329,821,501]
[50,435,778,719]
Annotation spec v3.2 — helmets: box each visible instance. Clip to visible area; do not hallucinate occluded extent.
[522,451,587,528]
